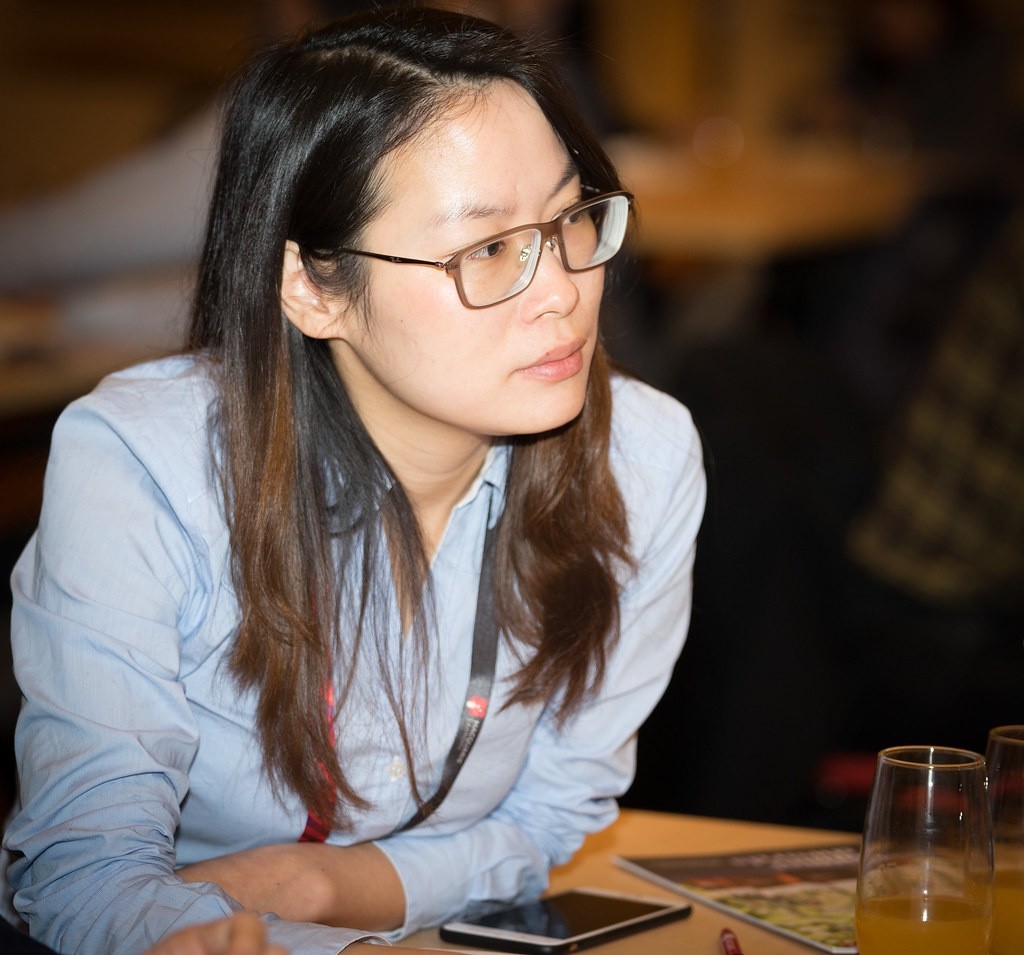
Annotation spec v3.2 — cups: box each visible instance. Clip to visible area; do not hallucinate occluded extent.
[984,726,1024,955]
[855,745,995,955]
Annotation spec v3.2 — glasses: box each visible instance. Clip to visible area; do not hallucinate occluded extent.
[329,183,634,310]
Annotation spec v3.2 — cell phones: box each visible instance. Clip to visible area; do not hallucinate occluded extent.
[438,887,692,955]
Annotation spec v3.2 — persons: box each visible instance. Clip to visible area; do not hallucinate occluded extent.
[0,1,705,955]
[827,0,1022,753]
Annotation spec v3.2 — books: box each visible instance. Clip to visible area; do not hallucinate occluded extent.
[611,842,862,955]
